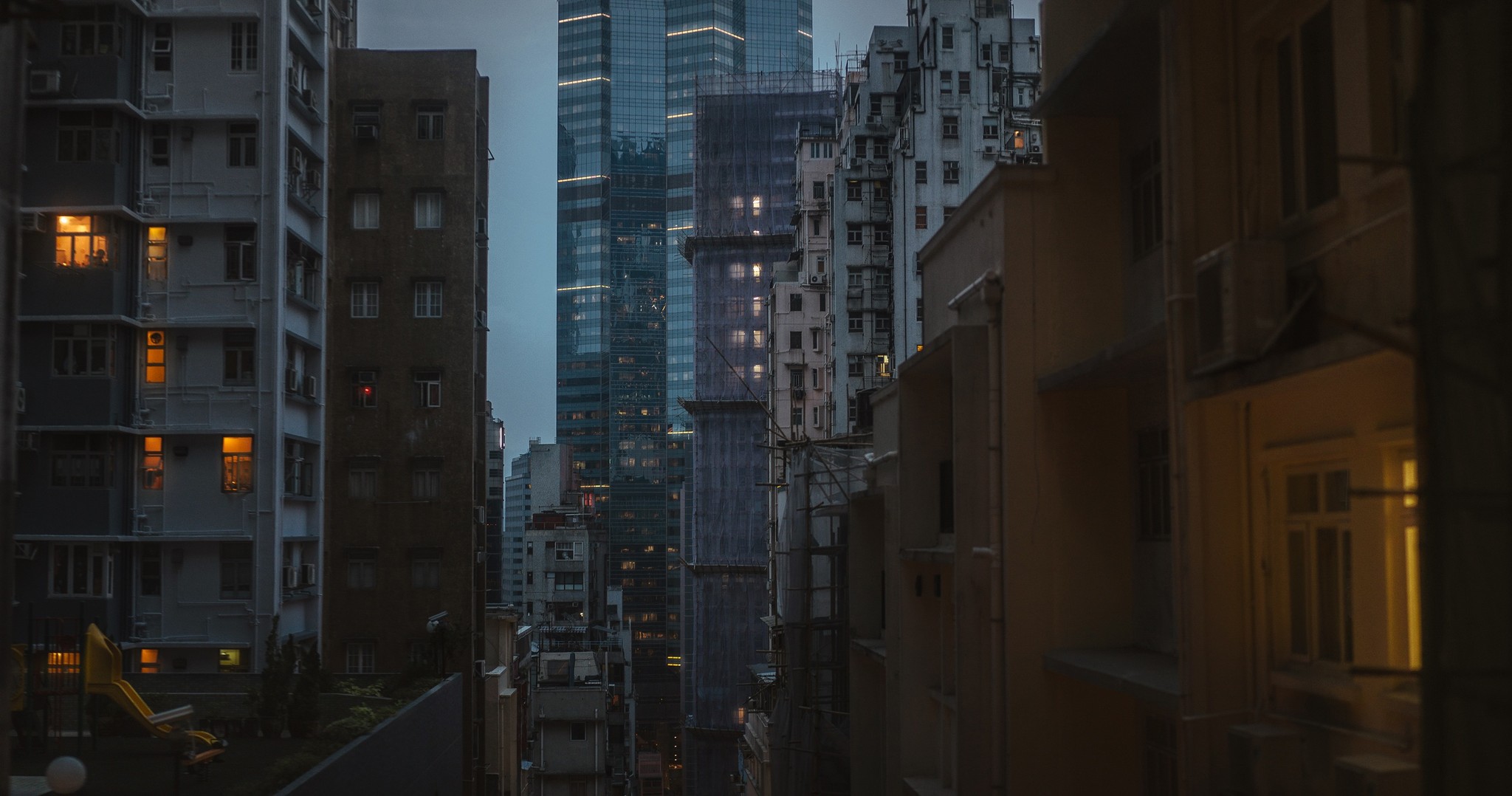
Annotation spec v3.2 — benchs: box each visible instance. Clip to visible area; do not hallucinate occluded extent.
[182,739,227,783]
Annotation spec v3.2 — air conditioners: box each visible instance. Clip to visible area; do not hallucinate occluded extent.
[864,115,882,125]
[304,375,316,398]
[849,157,862,166]
[476,218,488,241]
[286,368,299,392]
[14,387,25,413]
[1032,145,1043,153]
[1189,242,1289,369]
[14,543,39,560]
[305,170,321,190]
[21,212,47,233]
[810,274,823,284]
[306,0,322,12]
[30,70,60,93]
[1226,724,1303,796]
[283,565,299,589]
[303,89,317,112]
[301,564,316,585]
[288,67,299,95]
[1330,754,1423,796]
[474,505,485,525]
[288,147,302,174]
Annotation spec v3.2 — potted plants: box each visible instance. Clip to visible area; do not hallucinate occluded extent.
[199,612,325,739]
[86,690,162,737]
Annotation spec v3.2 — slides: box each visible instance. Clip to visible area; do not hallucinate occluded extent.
[86,623,216,745]
